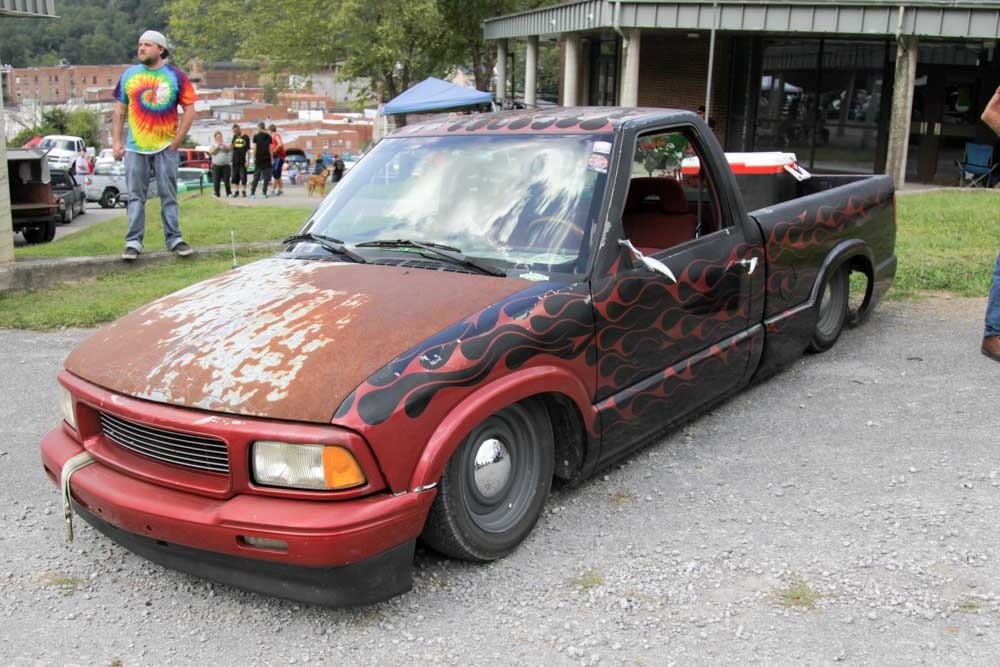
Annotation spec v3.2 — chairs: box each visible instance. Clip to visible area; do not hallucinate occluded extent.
[955,142,998,187]
[622,177,697,262]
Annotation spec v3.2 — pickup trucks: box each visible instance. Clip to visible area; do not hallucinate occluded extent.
[40,107,896,609]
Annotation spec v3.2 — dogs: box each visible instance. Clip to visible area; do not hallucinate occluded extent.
[308,169,330,198]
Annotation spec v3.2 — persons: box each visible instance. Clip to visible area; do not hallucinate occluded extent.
[981,85,1000,362]
[76,150,95,175]
[280,141,376,182]
[248,122,272,198]
[209,131,232,198]
[111,31,199,259]
[231,125,250,198]
[267,124,285,196]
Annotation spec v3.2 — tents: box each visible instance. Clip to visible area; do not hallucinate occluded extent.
[379,76,493,129]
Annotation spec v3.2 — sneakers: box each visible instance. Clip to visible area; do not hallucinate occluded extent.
[174,242,193,256]
[122,246,138,259]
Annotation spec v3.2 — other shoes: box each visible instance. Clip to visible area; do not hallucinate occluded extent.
[262,193,267,198]
[228,193,232,199]
[981,335,1000,361]
[242,192,247,198]
[268,191,274,198]
[234,191,239,198]
[213,193,221,198]
[250,194,256,200]
[276,190,283,196]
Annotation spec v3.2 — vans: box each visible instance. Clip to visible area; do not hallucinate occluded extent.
[19,135,212,224]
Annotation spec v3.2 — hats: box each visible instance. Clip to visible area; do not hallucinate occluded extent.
[139,30,170,59]
[267,124,276,131]
[80,147,86,152]
[257,122,265,129]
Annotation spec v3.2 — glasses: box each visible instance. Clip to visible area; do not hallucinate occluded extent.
[697,112,702,114]
